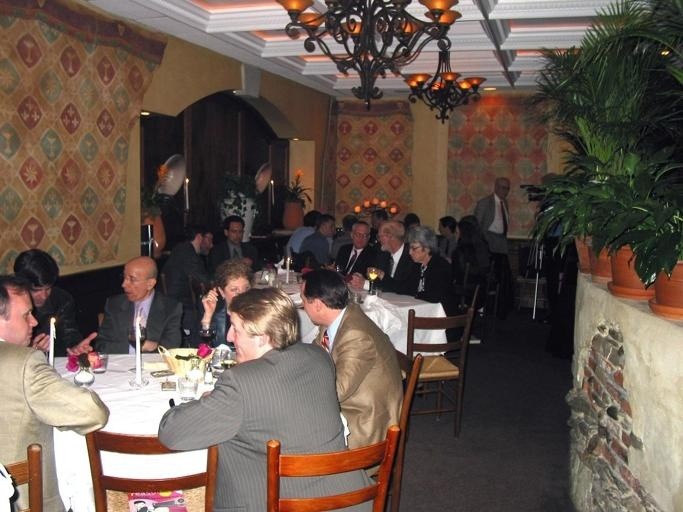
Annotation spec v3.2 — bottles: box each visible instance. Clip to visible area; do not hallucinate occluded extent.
[73,354,94,389]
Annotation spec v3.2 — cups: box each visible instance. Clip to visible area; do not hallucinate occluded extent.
[177,377,198,402]
[266,272,276,287]
[89,351,107,373]
[349,291,361,304]
[210,349,229,378]
[371,286,382,299]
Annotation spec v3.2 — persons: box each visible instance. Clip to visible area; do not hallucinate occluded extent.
[191,257,254,349]
[156,286,381,512]
[94,255,184,354]
[0,273,111,512]
[10,249,98,359]
[471,175,513,258]
[159,208,491,354]
[297,267,404,477]
[541,191,578,363]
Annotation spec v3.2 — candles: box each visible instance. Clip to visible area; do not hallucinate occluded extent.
[133,315,147,382]
[46,317,57,367]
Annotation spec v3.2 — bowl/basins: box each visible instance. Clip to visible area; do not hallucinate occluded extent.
[157,345,212,383]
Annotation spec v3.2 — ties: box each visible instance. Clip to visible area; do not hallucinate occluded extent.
[385,256,394,278]
[320,331,330,351]
[344,250,357,275]
[232,248,241,261]
[500,201,509,233]
[131,302,146,345]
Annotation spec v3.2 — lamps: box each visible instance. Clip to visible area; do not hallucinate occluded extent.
[274,1,465,109]
[351,195,400,219]
[396,50,489,125]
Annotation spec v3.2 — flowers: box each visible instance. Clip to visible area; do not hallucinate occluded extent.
[66,352,83,375]
[193,344,211,367]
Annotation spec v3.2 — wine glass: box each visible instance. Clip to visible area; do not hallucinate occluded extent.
[366,267,379,294]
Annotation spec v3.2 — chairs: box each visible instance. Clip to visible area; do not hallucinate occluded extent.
[264,425,400,511]
[384,336,424,511]
[82,427,220,511]
[0,440,43,512]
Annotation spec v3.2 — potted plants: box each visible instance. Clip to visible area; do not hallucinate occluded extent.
[218,169,258,244]
[137,186,179,253]
[278,169,311,228]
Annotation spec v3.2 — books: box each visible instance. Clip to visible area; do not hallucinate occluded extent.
[126,489,187,511]
